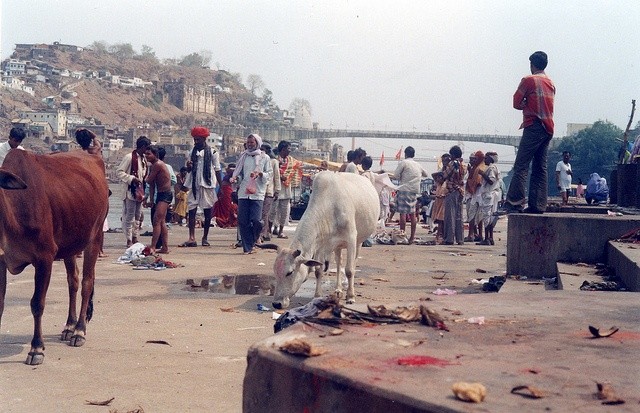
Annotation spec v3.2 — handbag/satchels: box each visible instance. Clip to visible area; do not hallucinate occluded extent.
[391,228,409,244]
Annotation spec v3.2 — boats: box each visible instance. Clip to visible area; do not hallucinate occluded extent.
[290,163,329,219]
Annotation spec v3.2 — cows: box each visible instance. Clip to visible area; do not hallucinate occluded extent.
[0,147,111,366]
[254,168,383,311]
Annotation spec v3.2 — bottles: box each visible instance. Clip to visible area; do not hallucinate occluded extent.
[232,178,238,193]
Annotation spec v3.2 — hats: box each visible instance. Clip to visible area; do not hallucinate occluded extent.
[432,172,445,181]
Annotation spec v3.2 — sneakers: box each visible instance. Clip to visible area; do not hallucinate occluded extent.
[439,241,454,245]
[278,232,287,239]
[524,208,544,214]
[492,202,524,216]
[474,238,483,242]
[491,241,494,245]
[458,242,464,245]
[476,240,490,245]
[464,237,474,242]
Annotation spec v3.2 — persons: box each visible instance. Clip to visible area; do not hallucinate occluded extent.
[476,152,500,245]
[178,126,222,248]
[389,145,428,240]
[229,133,273,254]
[75,127,109,259]
[173,168,187,224]
[339,149,355,173]
[143,148,177,251]
[215,163,239,228]
[576,177,584,197]
[116,135,151,249]
[0,126,26,170]
[275,140,327,239]
[345,147,367,175]
[360,155,373,180]
[464,151,488,242]
[416,145,465,245]
[491,50,556,215]
[257,140,281,244]
[556,151,573,206]
[465,152,478,239]
[142,144,174,255]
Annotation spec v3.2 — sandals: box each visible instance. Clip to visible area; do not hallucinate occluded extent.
[202,239,210,246]
[178,241,197,247]
